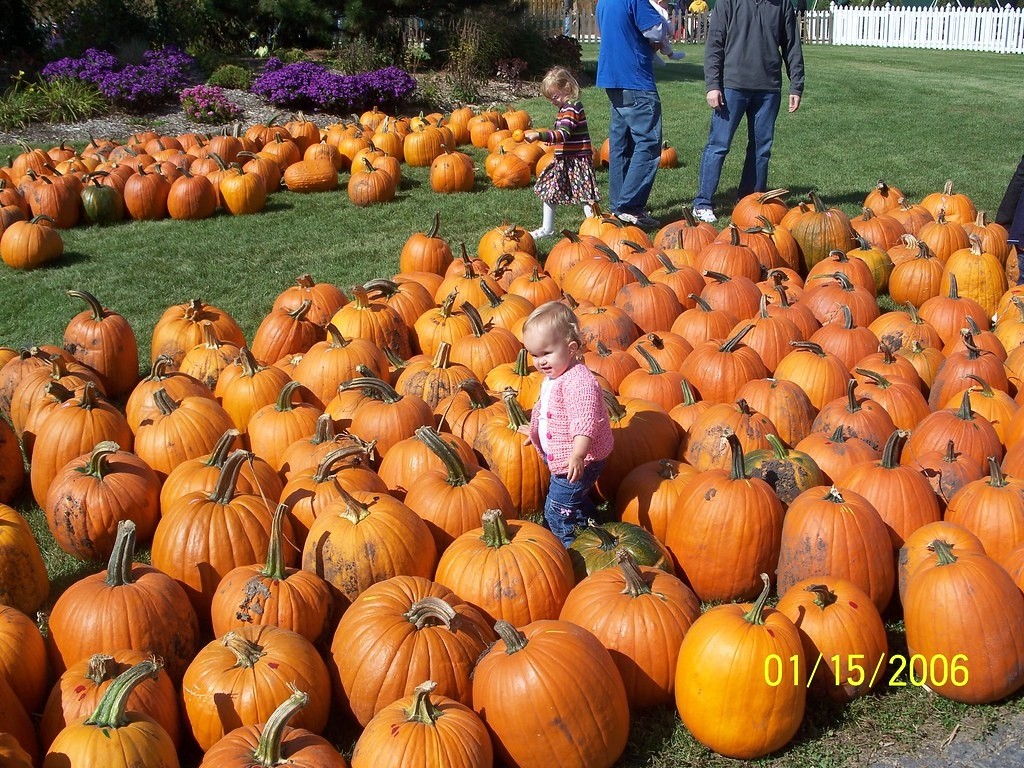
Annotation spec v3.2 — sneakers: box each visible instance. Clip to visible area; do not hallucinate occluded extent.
[691,205,718,222]
[529,228,557,241]
[612,211,660,229]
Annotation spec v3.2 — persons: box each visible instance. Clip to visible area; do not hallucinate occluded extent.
[692,0,805,223]
[524,67,602,239]
[995,155,1024,285]
[563,0,710,67]
[595,0,668,228]
[517,301,614,549]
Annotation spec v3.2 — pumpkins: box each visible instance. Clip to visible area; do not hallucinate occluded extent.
[0,176,1024,768]
[0,100,675,270]
